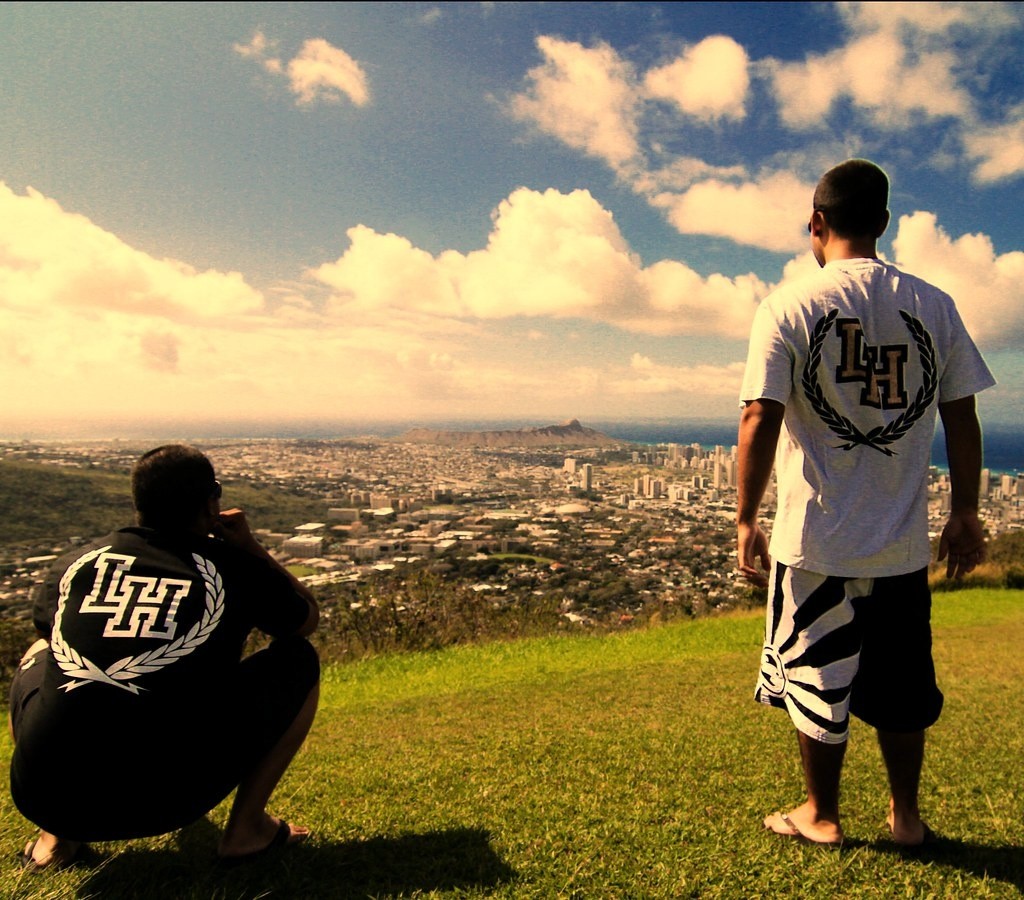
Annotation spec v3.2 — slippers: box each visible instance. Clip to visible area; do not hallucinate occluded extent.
[898,820,931,854]
[770,809,843,844]
[218,820,314,864]
[23,829,76,873]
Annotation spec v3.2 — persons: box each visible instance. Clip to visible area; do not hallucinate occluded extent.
[736,158,996,847]
[9,444,322,874]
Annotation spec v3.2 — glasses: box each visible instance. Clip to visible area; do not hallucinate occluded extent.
[196,481,222,508]
